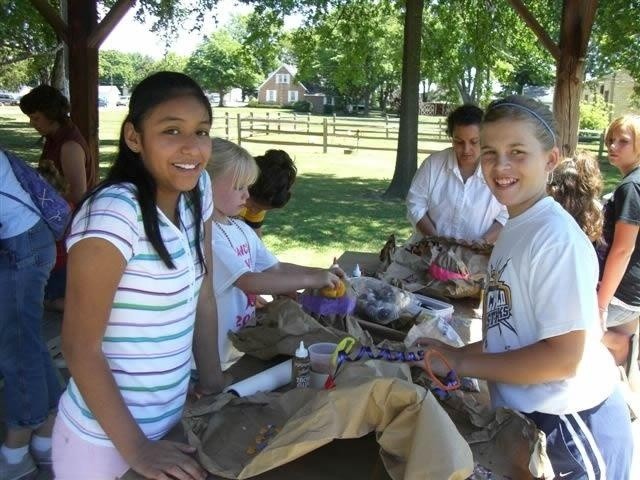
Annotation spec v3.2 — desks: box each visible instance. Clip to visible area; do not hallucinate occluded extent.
[120,250,542,478]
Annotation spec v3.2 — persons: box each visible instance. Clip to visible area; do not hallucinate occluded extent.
[188,137,346,375]
[587,113,640,373]
[403,103,513,248]
[230,146,300,311]
[0,143,69,480]
[404,93,640,479]
[50,71,234,480]
[17,81,99,372]
[543,145,629,352]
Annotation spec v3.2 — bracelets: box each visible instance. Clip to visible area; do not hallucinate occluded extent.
[595,305,608,313]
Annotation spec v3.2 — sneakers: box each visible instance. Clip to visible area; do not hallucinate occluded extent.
[0,451,39,480]
[29,447,51,470]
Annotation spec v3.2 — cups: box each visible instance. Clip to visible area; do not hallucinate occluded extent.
[307,342,339,376]
[309,370,330,390]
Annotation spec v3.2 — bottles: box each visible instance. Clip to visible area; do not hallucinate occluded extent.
[353,264,361,278]
[291,341,311,388]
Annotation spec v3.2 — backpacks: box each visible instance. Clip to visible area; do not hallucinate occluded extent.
[3,149,74,242]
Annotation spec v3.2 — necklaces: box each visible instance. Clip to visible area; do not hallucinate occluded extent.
[213,214,254,271]
